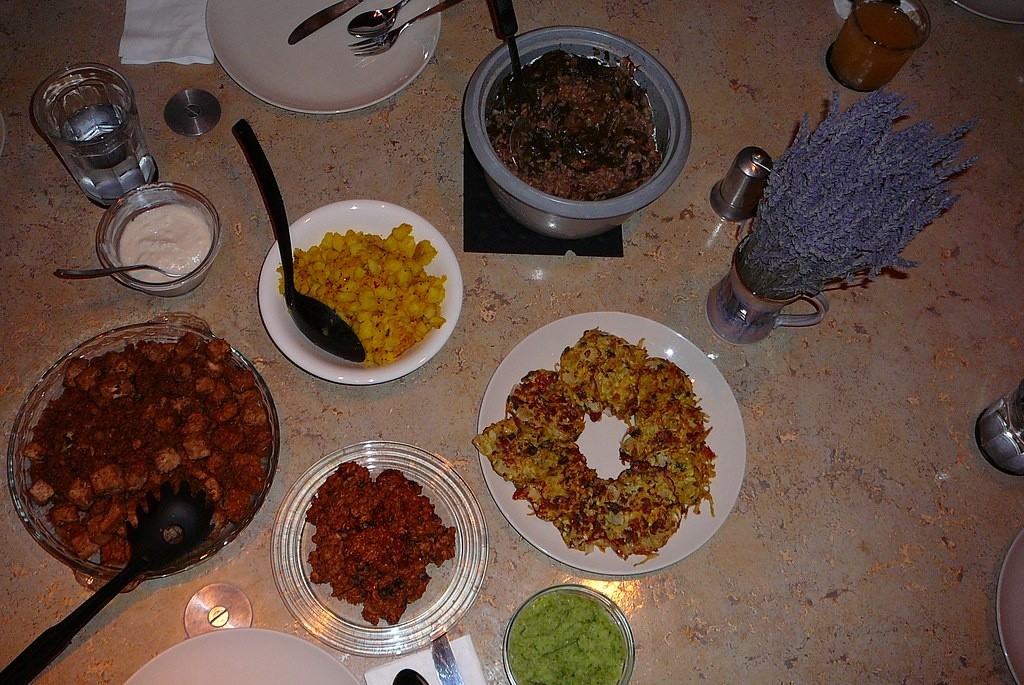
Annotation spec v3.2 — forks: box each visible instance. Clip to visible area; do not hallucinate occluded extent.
[348,0,465,56]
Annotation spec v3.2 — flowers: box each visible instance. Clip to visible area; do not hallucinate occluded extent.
[743,83,991,299]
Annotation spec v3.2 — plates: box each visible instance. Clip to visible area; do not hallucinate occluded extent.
[270,439,489,658]
[123,627,362,685]
[205,0,439,114]
[478,311,746,576]
[258,199,463,386]
[997,529,1024,685]
[503,584,635,685]
[834,0,926,29]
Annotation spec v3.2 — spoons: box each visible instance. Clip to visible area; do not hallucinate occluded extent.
[53,264,188,279]
[392,669,430,685]
[347,0,410,39]
[231,118,365,363]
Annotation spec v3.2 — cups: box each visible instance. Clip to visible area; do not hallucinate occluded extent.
[31,62,158,205]
[975,379,1024,476]
[825,0,931,93]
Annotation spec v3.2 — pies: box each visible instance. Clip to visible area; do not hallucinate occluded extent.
[470,324,716,564]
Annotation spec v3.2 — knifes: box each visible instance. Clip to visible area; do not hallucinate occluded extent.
[431,634,465,685]
[288,0,364,45]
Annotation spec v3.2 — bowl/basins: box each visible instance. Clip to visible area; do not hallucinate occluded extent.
[6,311,280,594]
[464,24,692,219]
[95,181,222,297]
